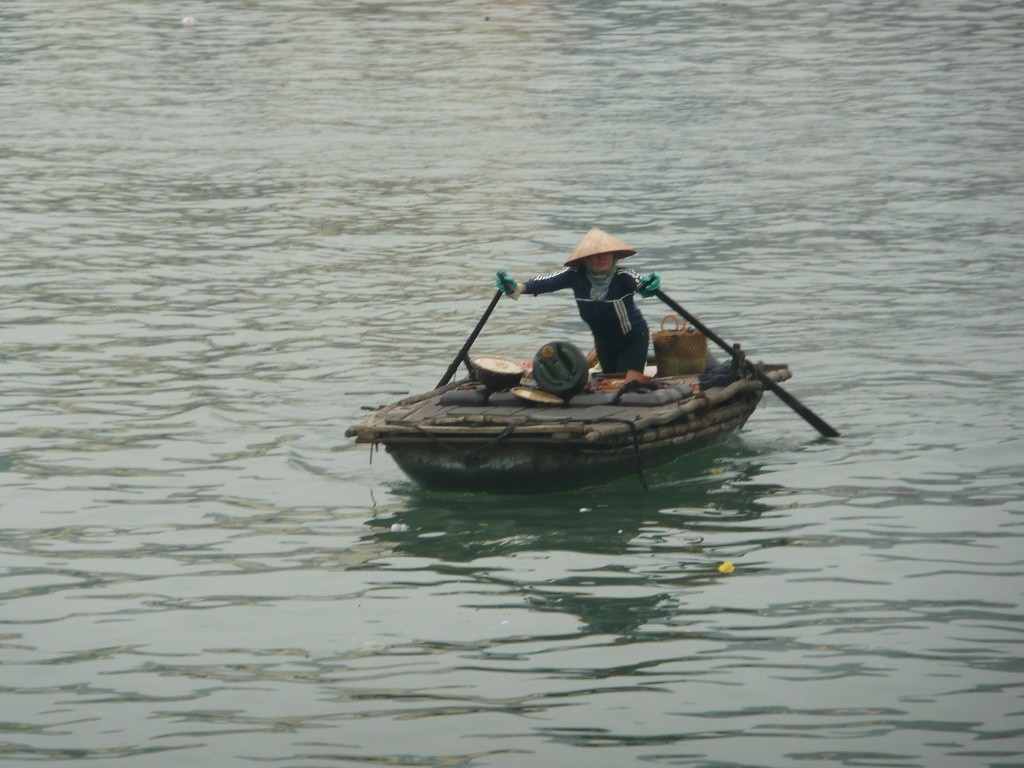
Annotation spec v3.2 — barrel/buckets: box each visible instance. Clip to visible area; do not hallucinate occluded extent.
[532,341,588,398]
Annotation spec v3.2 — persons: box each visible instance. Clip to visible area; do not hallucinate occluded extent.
[495,227,660,375]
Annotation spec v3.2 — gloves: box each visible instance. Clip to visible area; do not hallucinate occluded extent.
[637,275,661,299]
[494,270,523,301]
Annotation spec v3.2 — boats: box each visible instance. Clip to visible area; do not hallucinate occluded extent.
[344,272,841,493]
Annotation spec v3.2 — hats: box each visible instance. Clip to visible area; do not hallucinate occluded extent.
[563,226,637,269]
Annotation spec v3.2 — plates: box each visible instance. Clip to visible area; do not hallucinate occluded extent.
[511,386,566,407]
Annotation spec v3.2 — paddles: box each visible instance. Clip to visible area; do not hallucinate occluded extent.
[635,273,840,438]
[433,273,516,390]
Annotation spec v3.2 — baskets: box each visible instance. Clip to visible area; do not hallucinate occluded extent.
[652,315,708,377]
